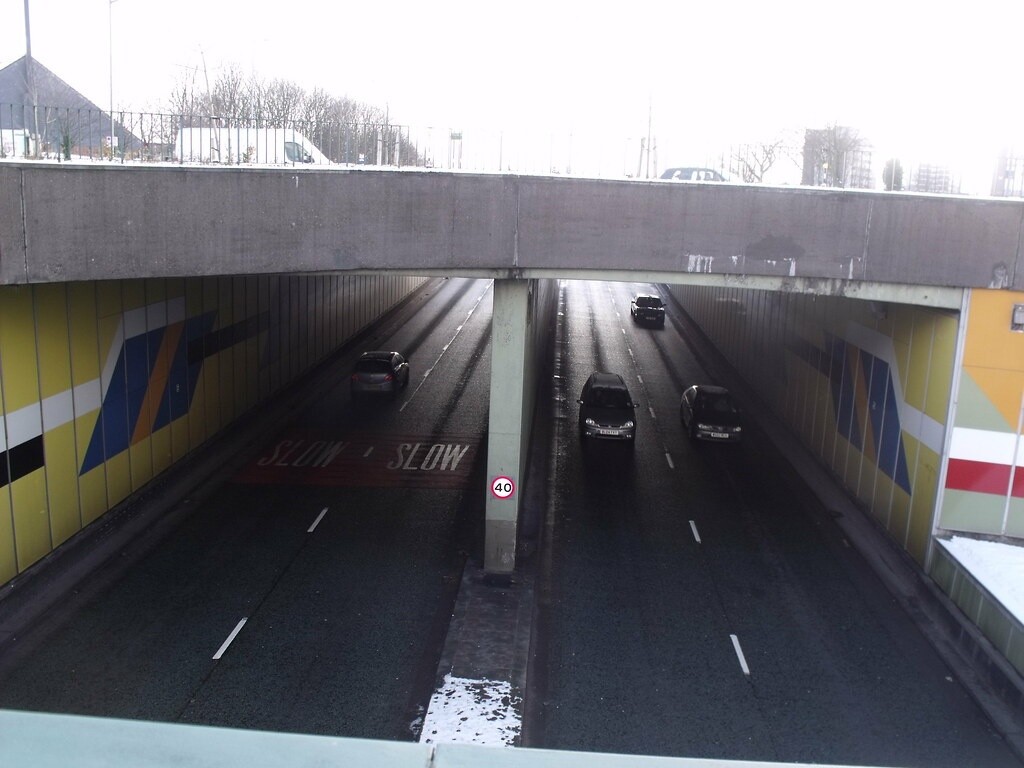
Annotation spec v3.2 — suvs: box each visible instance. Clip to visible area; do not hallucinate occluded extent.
[576,371,641,447]
[350,351,409,395]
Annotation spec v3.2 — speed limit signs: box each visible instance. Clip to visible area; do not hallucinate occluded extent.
[492,476,514,498]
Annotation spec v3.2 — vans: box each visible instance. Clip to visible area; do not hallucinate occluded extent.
[176,128,334,164]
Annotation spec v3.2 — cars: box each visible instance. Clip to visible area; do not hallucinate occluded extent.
[648,167,729,182]
[630,296,667,325]
[680,384,743,448]
